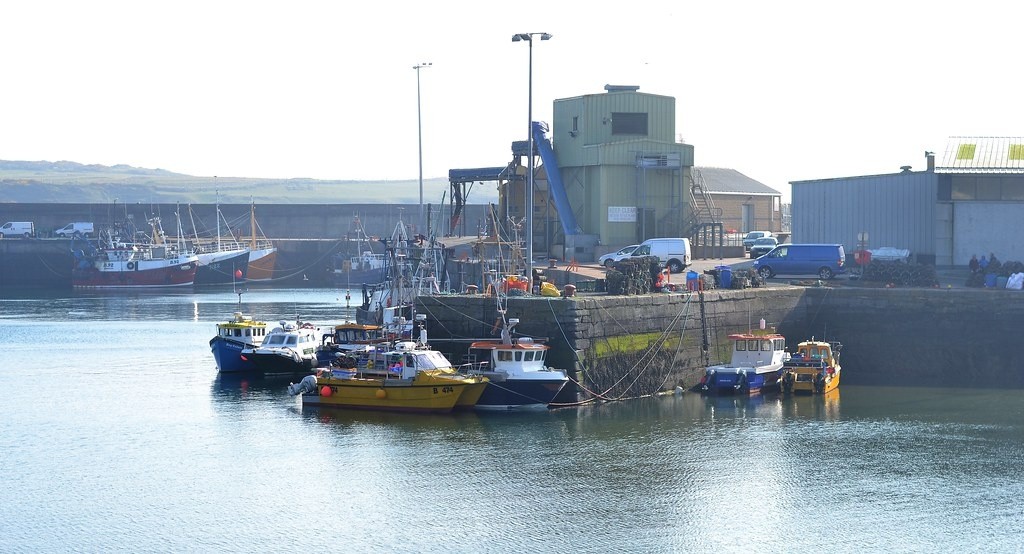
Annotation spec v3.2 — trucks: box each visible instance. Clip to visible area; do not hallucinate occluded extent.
[56,221,94,239]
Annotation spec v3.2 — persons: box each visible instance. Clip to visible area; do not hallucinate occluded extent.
[990,253,1001,267]
[34,228,48,238]
[969,255,979,271]
[980,256,989,267]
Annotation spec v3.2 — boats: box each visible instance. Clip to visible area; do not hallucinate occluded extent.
[287,208,571,415]
[251,296,333,376]
[69,176,277,288]
[701,304,791,396]
[208,260,270,373]
[777,322,842,394]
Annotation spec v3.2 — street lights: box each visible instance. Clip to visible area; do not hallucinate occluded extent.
[511,32,553,296]
[413,62,433,235]
[114,198,118,240]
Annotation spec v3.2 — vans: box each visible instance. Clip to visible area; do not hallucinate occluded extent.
[613,238,693,273]
[742,231,772,250]
[0,222,35,239]
[752,244,847,280]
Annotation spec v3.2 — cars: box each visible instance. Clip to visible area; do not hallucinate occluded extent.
[749,237,779,259]
[598,245,641,268]
